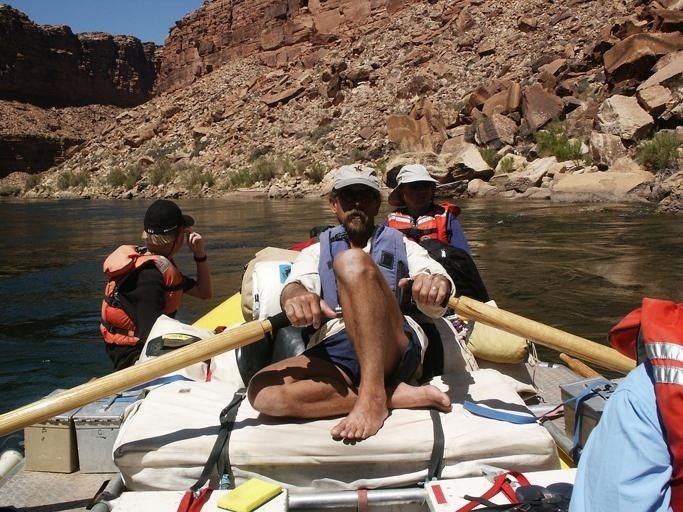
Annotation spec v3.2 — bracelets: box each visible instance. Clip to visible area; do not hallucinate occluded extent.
[193,254,208,263]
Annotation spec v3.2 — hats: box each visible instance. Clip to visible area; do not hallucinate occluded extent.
[387,164,438,207]
[330,163,381,193]
[143,199,194,235]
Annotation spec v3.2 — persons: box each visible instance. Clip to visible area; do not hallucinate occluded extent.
[246,164,452,440]
[381,164,472,256]
[100,200,215,375]
[565,296,682,512]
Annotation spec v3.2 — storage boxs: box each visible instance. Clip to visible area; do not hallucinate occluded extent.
[23,386,147,475]
[559,375,617,448]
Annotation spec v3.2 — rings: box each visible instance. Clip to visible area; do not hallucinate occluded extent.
[430,286,439,291]
